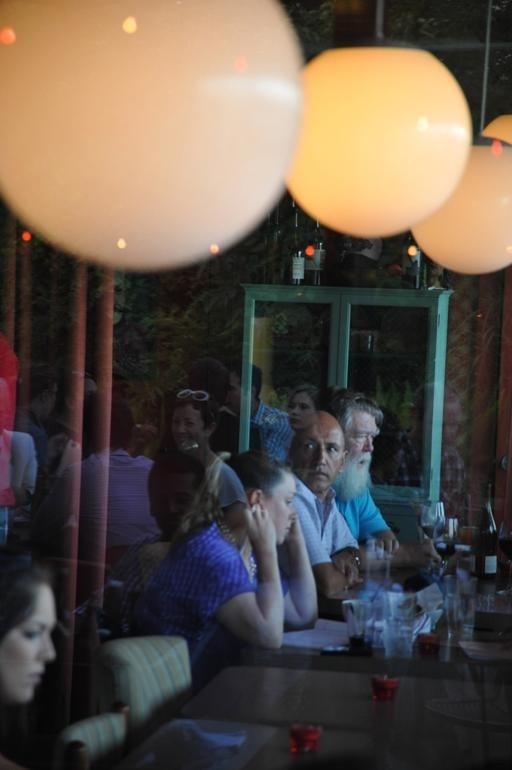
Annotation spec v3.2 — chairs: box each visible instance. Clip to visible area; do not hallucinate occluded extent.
[55,634,194,766]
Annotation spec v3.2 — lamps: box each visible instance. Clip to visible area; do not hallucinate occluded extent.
[287,0,472,238]
[2,1,304,275]
[411,0,512,274]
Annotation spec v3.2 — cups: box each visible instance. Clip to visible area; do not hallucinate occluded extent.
[441,550,495,634]
[342,599,381,648]
[381,590,430,658]
[419,501,446,573]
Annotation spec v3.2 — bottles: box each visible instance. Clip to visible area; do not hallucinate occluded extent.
[401,228,421,289]
[305,218,329,287]
[469,480,499,577]
[287,206,305,286]
[262,202,286,285]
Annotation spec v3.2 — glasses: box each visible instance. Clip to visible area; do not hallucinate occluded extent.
[175,387,210,402]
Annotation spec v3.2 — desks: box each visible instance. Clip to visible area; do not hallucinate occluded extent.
[122,542,511,768]
[122,542,512,770]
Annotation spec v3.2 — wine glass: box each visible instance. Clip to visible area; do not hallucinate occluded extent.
[433,518,460,579]
